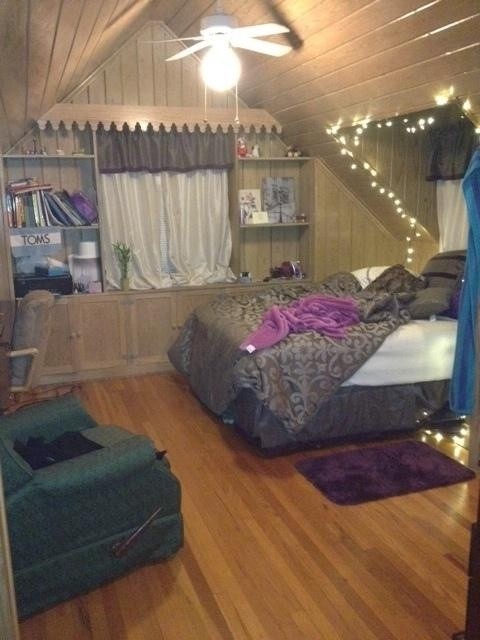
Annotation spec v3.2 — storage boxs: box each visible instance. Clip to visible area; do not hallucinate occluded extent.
[13,272,73,299]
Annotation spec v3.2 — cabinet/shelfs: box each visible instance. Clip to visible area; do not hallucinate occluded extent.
[14,286,227,387]
[1,126,108,304]
[224,132,312,281]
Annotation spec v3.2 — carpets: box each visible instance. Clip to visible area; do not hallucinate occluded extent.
[296,440,475,505]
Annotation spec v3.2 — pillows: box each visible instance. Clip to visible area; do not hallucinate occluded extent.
[404,250,466,318]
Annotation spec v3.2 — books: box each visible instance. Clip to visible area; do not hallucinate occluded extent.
[239,187,262,224]
[6,177,98,229]
[260,175,297,224]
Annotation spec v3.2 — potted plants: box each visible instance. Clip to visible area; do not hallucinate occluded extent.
[113,242,135,291]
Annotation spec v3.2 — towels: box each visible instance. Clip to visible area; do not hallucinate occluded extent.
[8,226,63,259]
[238,293,362,356]
[447,146,480,416]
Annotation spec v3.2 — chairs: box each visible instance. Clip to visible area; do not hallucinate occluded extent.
[1,289,53,414]
[1,393,184,623]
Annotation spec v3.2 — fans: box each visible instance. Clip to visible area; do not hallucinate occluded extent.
[141,0,292,64]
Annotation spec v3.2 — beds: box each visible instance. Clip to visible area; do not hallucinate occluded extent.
[171,290,460,451]
